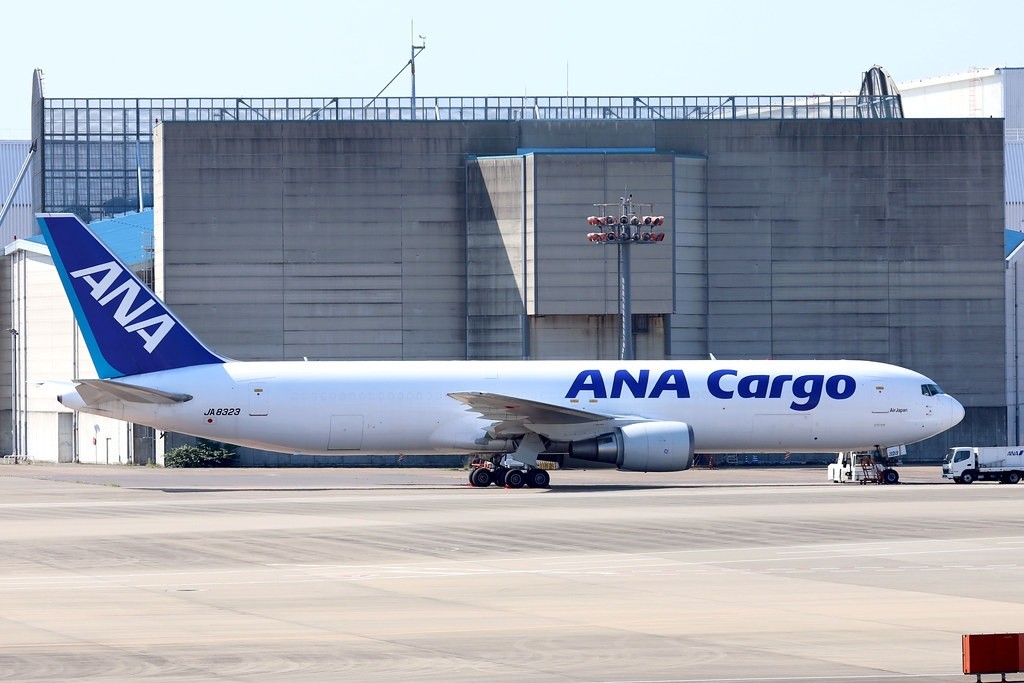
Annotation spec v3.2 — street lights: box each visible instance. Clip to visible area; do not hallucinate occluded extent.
[585,185,665,360]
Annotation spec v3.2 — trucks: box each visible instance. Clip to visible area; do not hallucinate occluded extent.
[942,446,1023,484]
[826,450,885,485]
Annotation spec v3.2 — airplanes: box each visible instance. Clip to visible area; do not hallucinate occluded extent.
[36,213,966,492]
